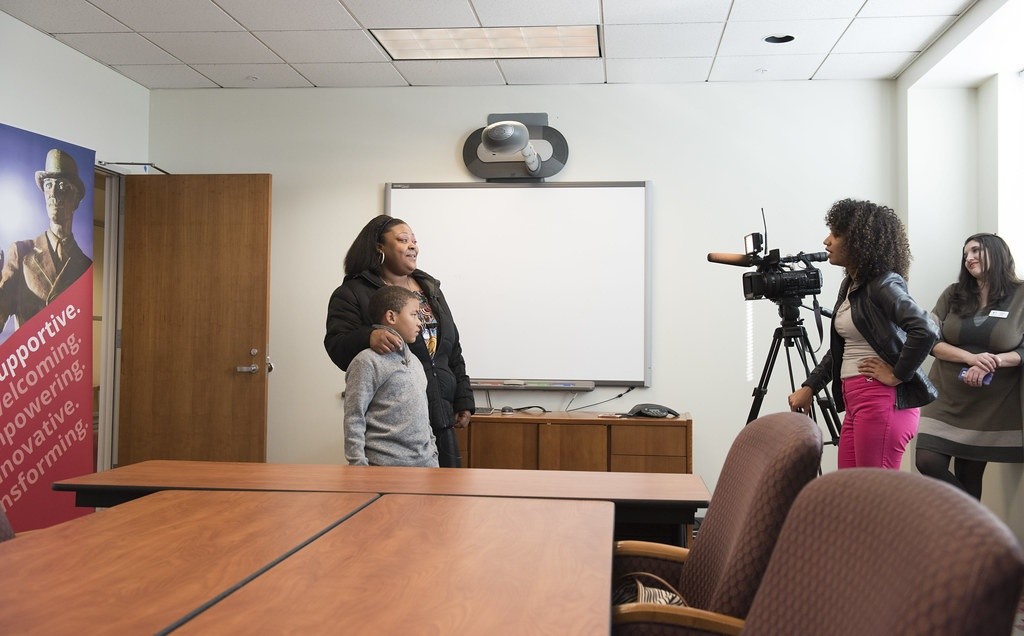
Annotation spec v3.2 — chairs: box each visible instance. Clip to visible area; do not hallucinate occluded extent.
[619,467,1024,636]
[614,411,824,619]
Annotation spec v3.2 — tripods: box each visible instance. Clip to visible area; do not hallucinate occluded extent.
[746,296,843,479]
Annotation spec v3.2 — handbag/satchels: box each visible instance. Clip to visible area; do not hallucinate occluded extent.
[611,571,689,607]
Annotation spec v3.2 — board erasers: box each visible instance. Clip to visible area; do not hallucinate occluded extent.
[504,382,526,385]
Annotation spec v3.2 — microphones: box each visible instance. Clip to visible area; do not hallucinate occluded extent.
[707,253,761,267]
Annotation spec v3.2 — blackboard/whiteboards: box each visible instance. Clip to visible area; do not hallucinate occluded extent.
[381,180,654,391]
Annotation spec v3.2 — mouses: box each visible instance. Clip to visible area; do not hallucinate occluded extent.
[502,406,514,415]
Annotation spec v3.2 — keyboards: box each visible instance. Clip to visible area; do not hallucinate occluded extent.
[474,407,495,415]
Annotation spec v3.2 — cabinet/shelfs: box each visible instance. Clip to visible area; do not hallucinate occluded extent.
[455,410,693,549]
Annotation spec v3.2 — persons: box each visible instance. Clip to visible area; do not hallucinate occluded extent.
[915,233,1024,501]
[343,286,439,467]
[789,199,941,470]
[0,148,93,335]
[324,214,475,468]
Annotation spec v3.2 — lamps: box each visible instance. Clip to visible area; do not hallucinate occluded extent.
[463,120,569,178]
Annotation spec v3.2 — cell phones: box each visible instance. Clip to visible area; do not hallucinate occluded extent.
[598,414,622,418]
[958,367,993,386]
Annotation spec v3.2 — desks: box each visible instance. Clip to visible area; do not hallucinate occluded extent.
[170,494,616,636]
[51,460,712,548]
[0,489,381,636]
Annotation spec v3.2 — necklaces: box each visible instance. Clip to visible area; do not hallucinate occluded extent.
[380,275,412,293]
